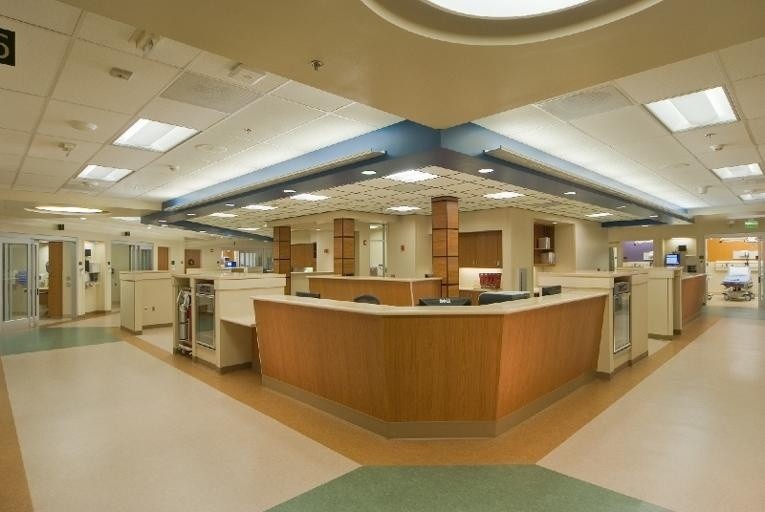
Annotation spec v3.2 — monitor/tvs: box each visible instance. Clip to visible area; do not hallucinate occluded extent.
[295,291,321,298]
[419,298,472,305]
[665,253,679,265]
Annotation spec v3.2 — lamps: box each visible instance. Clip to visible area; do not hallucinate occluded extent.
[65,116,100,135]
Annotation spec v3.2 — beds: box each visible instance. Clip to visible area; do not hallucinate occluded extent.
[719,264,756,301]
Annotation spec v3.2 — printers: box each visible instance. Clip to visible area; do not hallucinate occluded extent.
[478,291,530,304]
